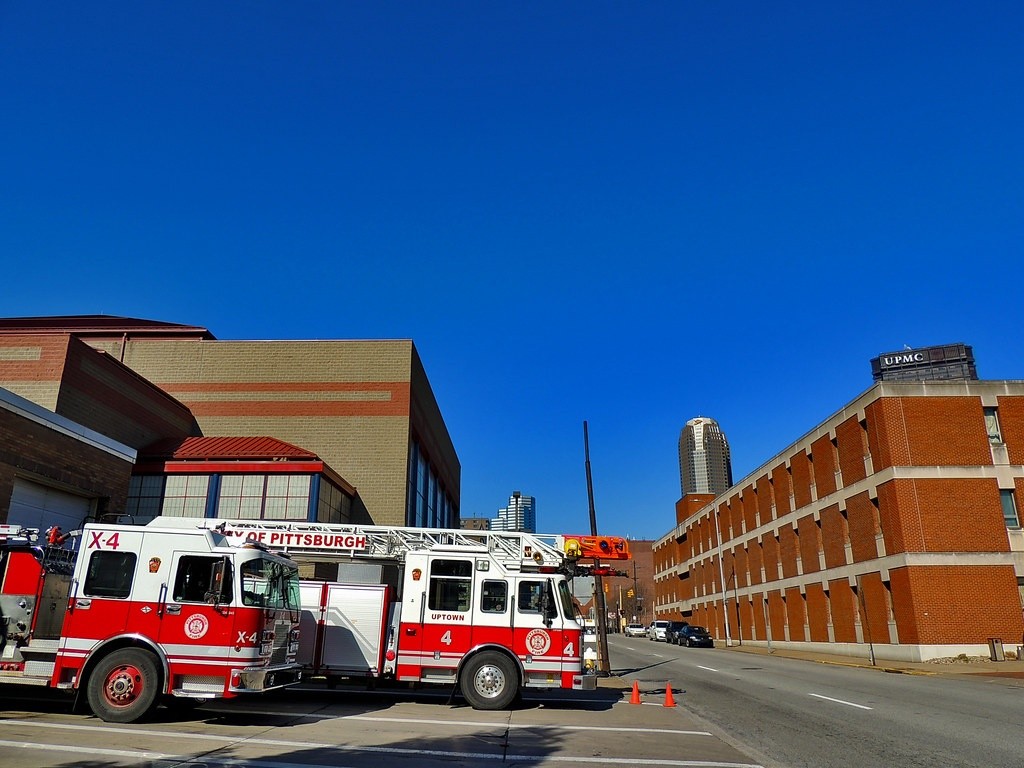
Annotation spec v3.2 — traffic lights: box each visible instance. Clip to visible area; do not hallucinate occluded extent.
[629,589,633,596]
[627,592,631,597]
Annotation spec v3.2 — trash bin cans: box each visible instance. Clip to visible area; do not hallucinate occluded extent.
[987,637,1006,661]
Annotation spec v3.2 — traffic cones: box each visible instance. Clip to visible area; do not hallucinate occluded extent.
[629,680,642,704]
[662,682,676,706]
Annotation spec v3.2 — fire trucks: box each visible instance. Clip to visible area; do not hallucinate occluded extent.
[147,516,632,711]
[0,522,302,723]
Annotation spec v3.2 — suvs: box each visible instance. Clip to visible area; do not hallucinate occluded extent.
[649,621,670,642]
[665,620,690,644]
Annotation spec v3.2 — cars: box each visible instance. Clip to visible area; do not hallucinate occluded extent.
[625,623,646,637]
[677,625,714,648]
